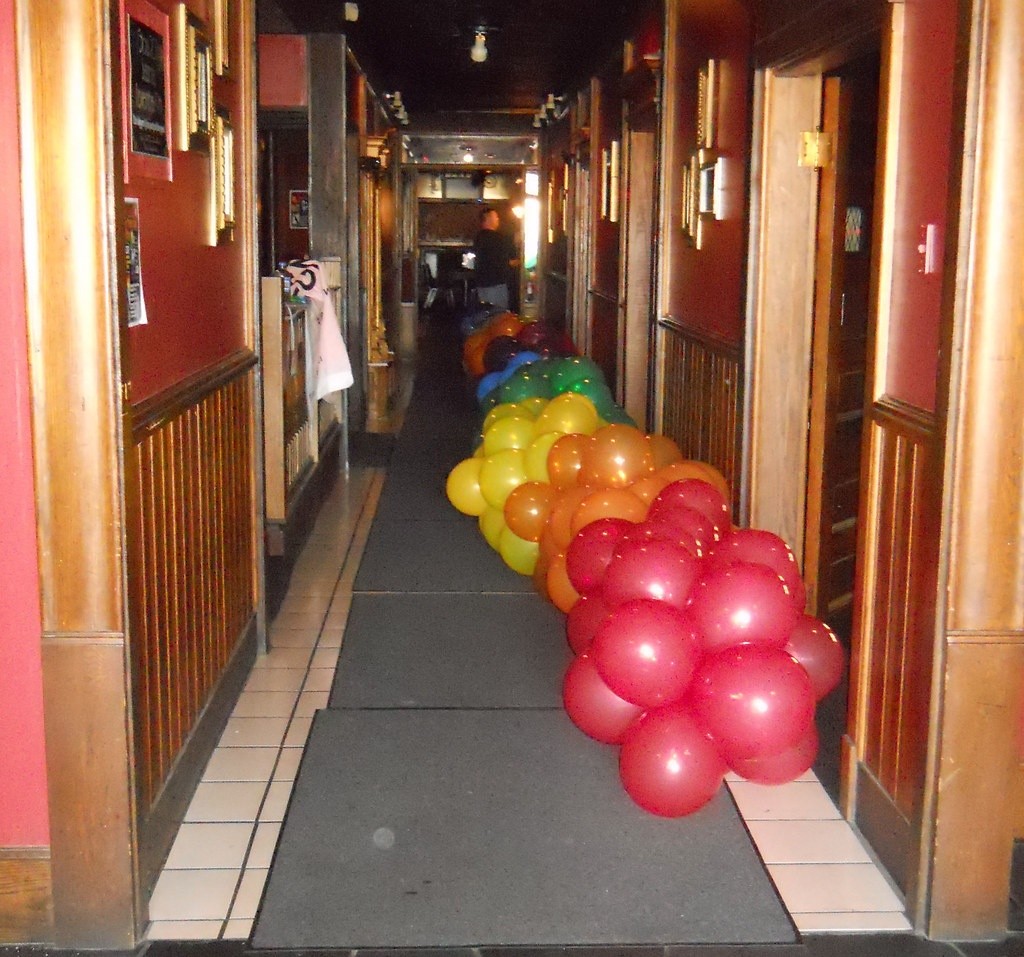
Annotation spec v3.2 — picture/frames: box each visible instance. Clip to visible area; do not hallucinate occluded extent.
[289,190,309,230]
[178,1,237,246]
[117,0,175,188]
[546,59,721,248]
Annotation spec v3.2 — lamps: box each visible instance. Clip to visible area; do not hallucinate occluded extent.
[469,31,488,62]
[395,112,409,124]
[547,94,562,108]
[385,91,402,106]
[539,104,549,119]
[533,113,542,127]
[390,104,405,119]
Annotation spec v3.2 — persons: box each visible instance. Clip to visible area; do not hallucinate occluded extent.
[474,208,519,308]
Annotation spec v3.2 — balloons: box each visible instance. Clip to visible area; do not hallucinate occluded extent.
[447,305,843,816]
